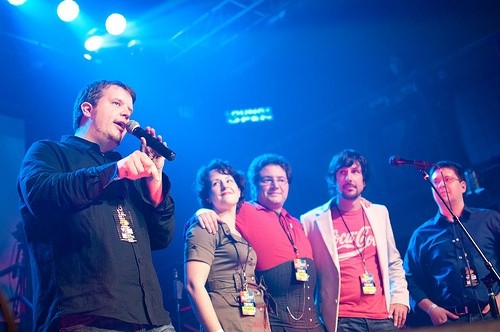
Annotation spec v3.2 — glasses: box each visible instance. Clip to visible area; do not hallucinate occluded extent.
[433,177,461,183]
[258,177,289,187]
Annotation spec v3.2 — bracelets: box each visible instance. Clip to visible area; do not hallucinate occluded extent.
[427,303,438,316]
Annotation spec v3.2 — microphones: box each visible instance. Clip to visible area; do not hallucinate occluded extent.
[126,120,176,161]
[388,155,436,167]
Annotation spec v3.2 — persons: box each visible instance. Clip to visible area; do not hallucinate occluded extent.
[300,149,410,332]
[17,80,176,332]
[185,154,372,332]
[403,161,500,329]
[183,158,271,332]
